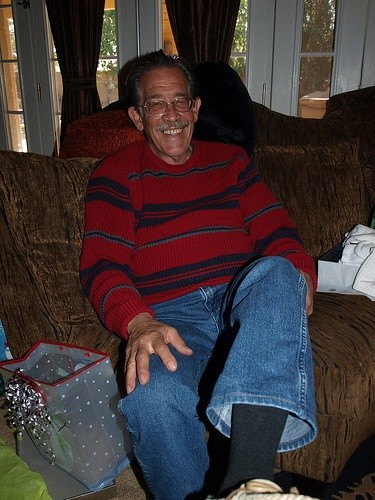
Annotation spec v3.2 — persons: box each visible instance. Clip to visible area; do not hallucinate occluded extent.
[79,48,321,500]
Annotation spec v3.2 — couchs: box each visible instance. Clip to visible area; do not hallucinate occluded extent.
[0,86,374,500]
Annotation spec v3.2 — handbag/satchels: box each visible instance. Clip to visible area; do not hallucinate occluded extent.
[0,339,135,493]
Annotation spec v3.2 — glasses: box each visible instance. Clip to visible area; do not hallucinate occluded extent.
[138,97,196,117]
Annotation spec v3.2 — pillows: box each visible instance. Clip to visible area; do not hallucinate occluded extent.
[0,150,117,330]
[325,86,375,209]
[256,135,368,264]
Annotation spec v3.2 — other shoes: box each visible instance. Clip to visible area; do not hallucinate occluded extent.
[205,478,320,500]
